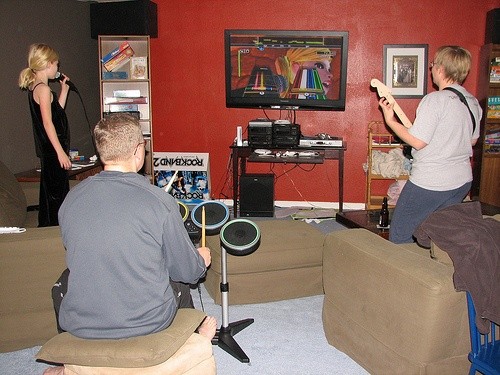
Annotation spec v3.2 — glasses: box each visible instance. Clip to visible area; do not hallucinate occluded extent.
[429,61,437,66]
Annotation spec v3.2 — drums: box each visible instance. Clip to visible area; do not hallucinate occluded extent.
[178,200,261,257]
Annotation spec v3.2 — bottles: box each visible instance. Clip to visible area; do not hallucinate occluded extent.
[379,197,389,227]
[68,149,78,161]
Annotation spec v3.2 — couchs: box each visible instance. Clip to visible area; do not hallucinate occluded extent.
[320,205,500,375]
[0,161,67,355]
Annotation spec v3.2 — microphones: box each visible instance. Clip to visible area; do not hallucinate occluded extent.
[55,72,78,92]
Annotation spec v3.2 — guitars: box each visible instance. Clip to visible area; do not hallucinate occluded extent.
[369,78,414,161]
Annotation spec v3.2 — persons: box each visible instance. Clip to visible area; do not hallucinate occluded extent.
[18,44,70,227]
[51,112,217,341]
[378,46,483,244]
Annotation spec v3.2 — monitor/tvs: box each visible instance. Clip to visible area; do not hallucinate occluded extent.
[224,29,348,112]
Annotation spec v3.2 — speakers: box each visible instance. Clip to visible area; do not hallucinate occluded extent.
[239,173,275,217]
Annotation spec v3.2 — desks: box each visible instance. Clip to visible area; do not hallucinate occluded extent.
[228,145,347,218]
[13,159,102,185]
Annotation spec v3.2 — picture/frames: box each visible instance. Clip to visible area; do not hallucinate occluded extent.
[382,44,427,98]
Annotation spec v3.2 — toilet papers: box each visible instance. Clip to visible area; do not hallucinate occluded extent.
[236,126,242,146]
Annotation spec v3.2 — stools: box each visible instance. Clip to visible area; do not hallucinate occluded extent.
[34,308,216,375]
[198,218,326,306]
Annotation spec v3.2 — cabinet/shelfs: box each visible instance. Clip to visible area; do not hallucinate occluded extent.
[97,34,154,185]
[474,42,500,206]
[365,120,412,210]
